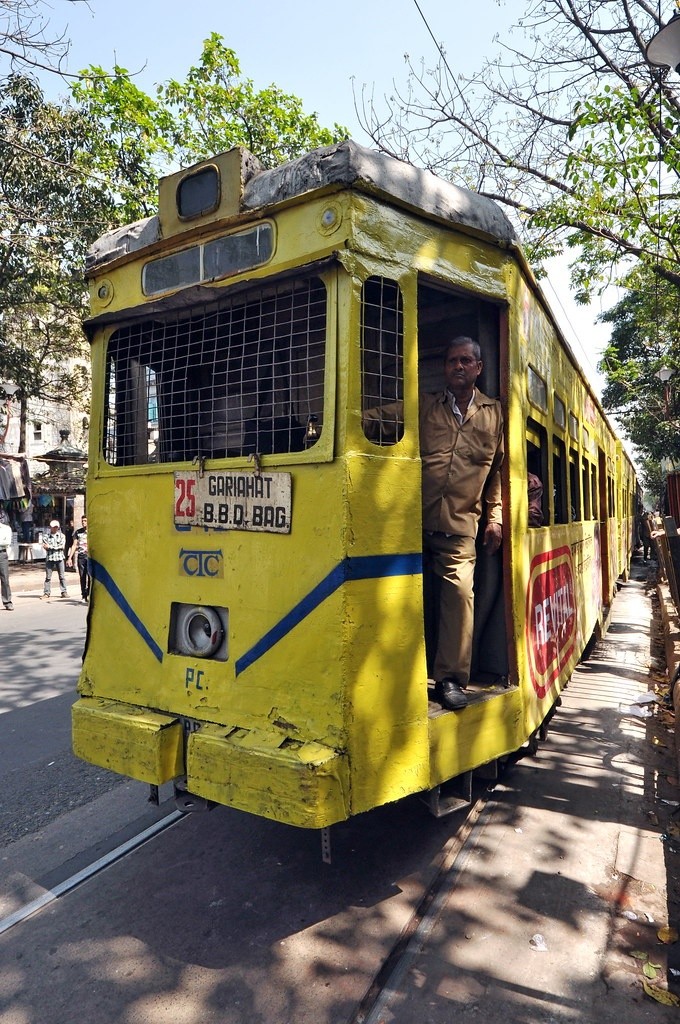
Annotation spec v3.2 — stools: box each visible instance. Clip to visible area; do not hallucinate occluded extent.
[17,545,34,566]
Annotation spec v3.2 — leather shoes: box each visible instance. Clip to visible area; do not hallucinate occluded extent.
[435,677,468,709]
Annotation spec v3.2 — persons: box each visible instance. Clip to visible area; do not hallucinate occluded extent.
[362,337,505,711]
[0,514,15,611]
[66,515,90,603]
[641,509,659,561]
[40,520,70,600]
[19,506,33,542]
[528,471,544,528]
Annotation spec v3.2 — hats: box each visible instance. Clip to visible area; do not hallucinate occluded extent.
[49,520,60,528]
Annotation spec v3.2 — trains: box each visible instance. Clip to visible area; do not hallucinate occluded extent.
[73,137,642,863]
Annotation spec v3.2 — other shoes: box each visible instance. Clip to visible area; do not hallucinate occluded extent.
[62,592,70,598]
[5,605,14,610]
[80,598,87,603]
[40,593,50,599]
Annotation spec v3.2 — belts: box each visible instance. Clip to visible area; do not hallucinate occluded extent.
[0,549,6,553]
